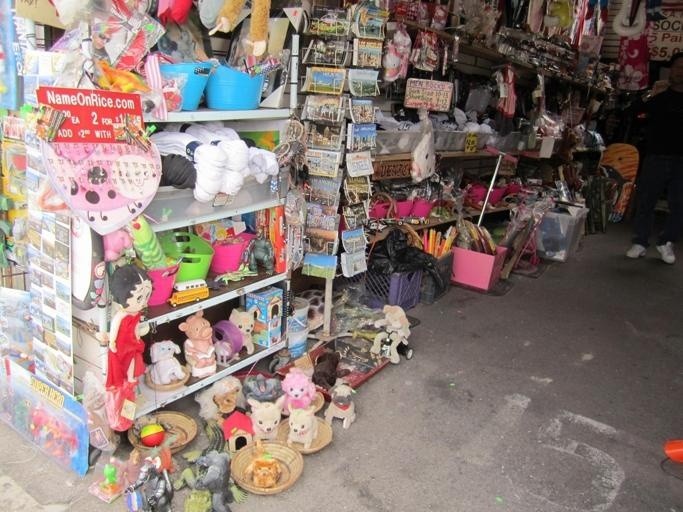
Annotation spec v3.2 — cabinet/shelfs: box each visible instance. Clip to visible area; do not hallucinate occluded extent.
[307,3,605,255]
[5,100,292,434]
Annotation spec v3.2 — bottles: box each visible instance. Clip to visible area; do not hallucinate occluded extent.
[497,35,549,70]
[463,132,477,153]
[586,59,593,79]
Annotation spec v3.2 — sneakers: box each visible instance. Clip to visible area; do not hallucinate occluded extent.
[625,243,647,259]
[656,242,677,265]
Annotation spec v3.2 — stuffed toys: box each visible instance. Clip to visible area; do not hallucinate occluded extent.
[370,305,410,364]
[144,307,255,385]
[246,347,357,448]
[383,30,411,80]
[73,213,357,512]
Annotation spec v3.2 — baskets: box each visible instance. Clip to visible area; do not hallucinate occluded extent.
[331,242,454,314]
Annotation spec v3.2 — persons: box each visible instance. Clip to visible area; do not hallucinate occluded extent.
[624,52,682,265]
[105,264,151,431]
